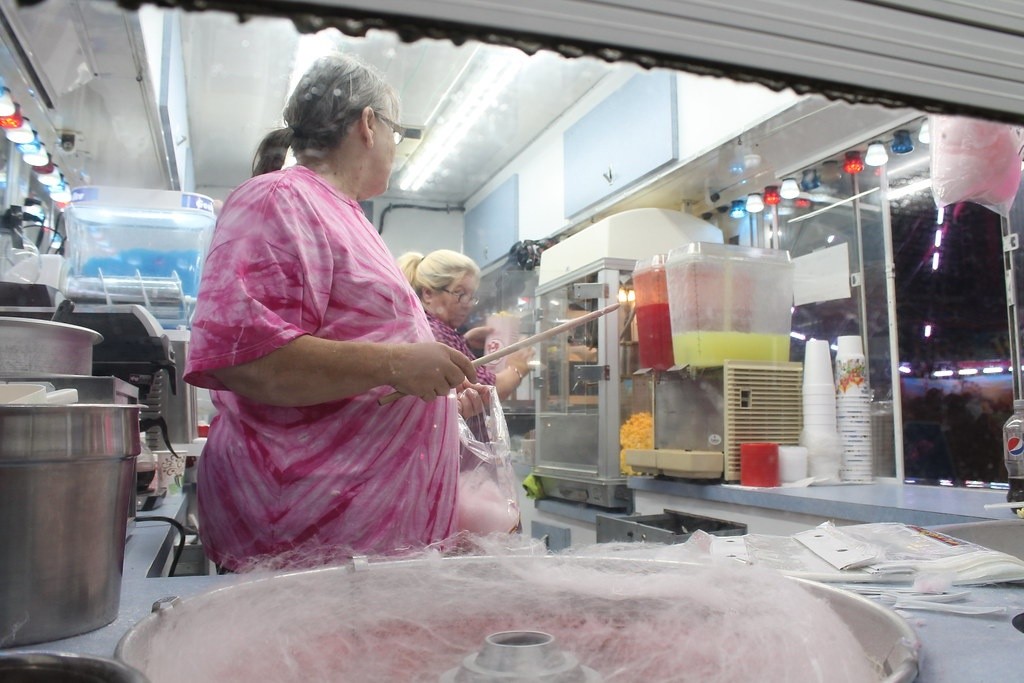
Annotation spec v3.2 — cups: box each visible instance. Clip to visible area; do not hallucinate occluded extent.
[520,439,535,464]
[150,450,186,499]
[740,335,872,487]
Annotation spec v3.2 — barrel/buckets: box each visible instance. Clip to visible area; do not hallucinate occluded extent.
[0,405,139,646]
[0,654,153,683]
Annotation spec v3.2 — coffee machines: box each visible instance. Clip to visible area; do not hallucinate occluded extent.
[0,304,176,510]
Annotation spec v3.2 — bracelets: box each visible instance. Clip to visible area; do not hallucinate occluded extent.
[509,366,523,382]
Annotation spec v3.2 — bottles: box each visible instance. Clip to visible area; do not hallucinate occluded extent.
[1003,399,1024,514]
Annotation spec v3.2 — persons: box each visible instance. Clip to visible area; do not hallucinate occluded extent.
[398,248,537,473]
[181,57,492,575]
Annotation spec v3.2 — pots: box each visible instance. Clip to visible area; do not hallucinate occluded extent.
[0,317,105,376]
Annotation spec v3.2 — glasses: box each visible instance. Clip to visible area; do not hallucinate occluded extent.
[441,287,480,306]
[350,108,406,146]
